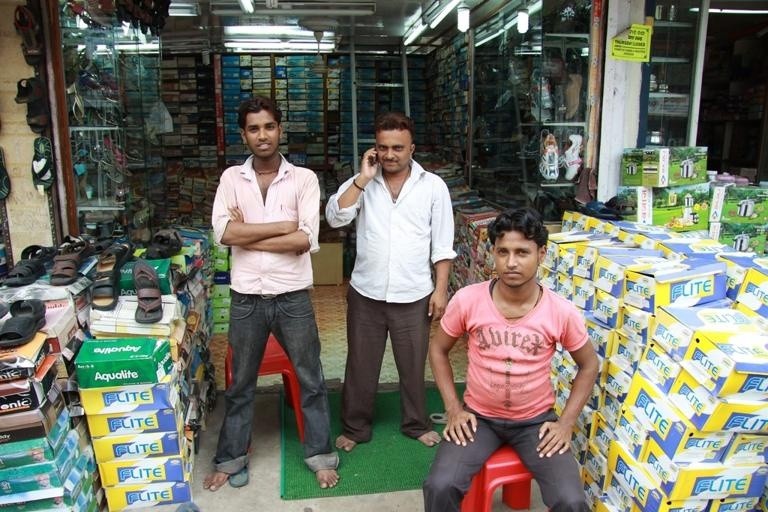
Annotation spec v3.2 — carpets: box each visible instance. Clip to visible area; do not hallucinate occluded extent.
[279,382,479,501]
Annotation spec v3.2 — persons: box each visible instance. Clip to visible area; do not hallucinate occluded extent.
[324,110,459,453]
[422,211,602,512]
[200,93,342,492]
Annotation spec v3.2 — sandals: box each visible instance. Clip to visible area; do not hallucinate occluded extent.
[586,193,637,220]
[14,3,46,65]
[15,77,50,133]
[60,0,171,239]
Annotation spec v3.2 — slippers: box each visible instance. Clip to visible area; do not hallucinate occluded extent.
[0,298,46,347]
[133,259,163,324]
[91,240,136,310]
[4,244,57,286]
[51,240,95,286]
[31,136,55,191]
[144,229,183,260]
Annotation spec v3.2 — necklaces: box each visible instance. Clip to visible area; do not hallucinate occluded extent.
[490,277,542,320]
[383,168,411,203]
[253,164,279,177]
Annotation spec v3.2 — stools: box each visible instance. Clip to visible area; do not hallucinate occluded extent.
[223,336,305,444]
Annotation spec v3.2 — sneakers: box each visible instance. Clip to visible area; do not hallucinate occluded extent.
[472,2,592,219]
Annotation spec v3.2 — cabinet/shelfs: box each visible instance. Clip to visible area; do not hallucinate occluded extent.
[532,29,594,189]
[642,0,711,159]
[56,45,126,213]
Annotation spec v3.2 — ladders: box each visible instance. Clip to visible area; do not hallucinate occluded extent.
[350,46,410,175]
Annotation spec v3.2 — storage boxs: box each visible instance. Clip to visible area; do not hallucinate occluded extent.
[451,146,768,512]
[119,50,474,222]
[1,229,229,510]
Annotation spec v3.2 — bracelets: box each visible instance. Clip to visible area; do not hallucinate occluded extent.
[353,178,365,193]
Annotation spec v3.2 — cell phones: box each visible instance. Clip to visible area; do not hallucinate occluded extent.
[369,151,379,166]
